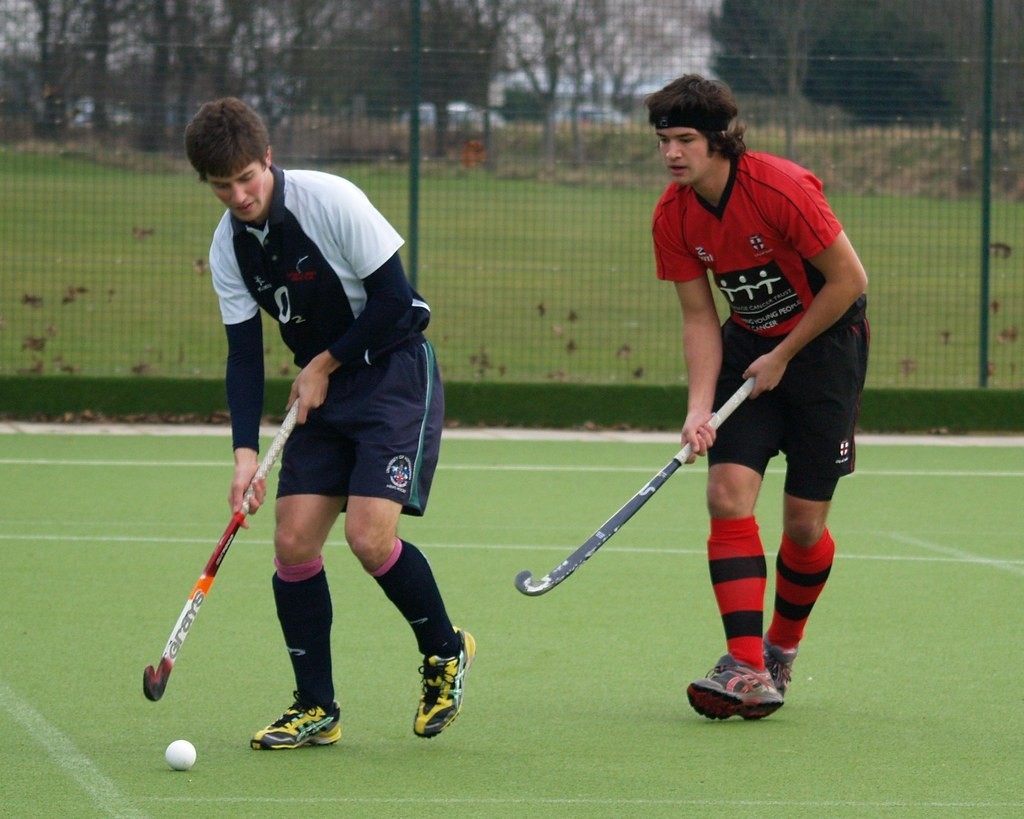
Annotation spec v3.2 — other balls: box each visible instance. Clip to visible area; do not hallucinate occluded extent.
[164,739,196,771]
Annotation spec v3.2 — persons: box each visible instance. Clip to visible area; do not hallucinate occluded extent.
[647,74,870,720]
[183,97,476,750]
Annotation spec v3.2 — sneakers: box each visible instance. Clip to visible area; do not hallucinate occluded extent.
[763,632,799,696]
[412,626,476,738]
[688,654,785,720]
[250,689,342,751]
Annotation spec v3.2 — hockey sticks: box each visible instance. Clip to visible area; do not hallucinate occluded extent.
[140,398,302,704]
[512,376,754,598]
